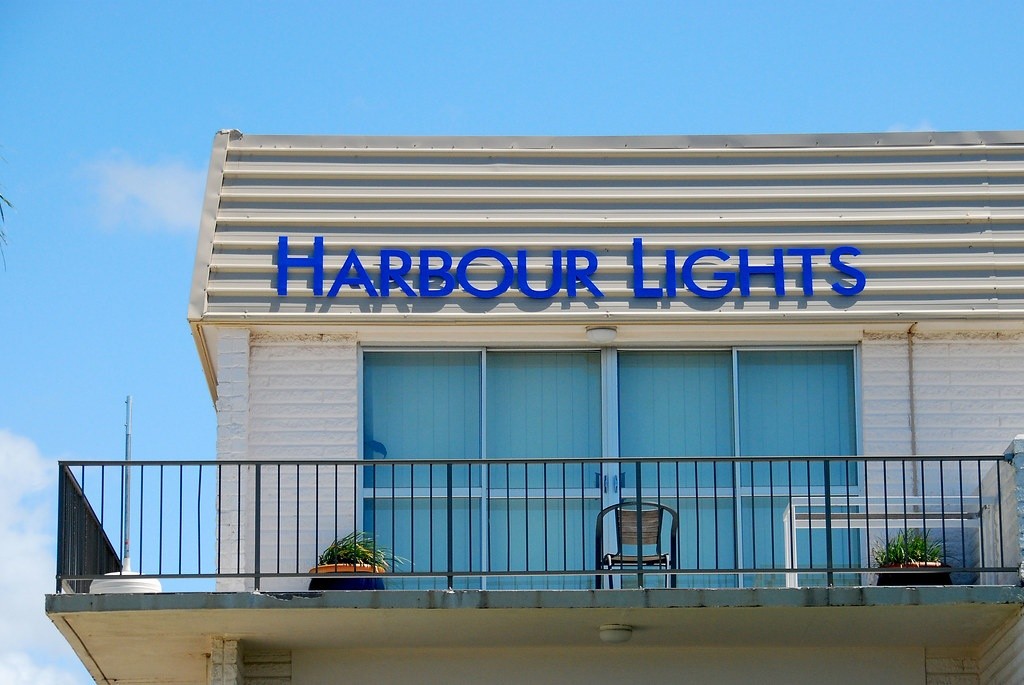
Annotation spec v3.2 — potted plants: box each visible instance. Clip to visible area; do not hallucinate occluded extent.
[308,530,415,590]
[862,527,965,586]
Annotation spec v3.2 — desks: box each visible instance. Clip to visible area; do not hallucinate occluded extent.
[782,498,998,588]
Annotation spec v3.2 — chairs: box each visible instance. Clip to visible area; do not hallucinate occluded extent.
[596,501,678,588]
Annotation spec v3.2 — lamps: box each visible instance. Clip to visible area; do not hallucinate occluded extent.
[585,325,617,343]
[600,625,633,642]
